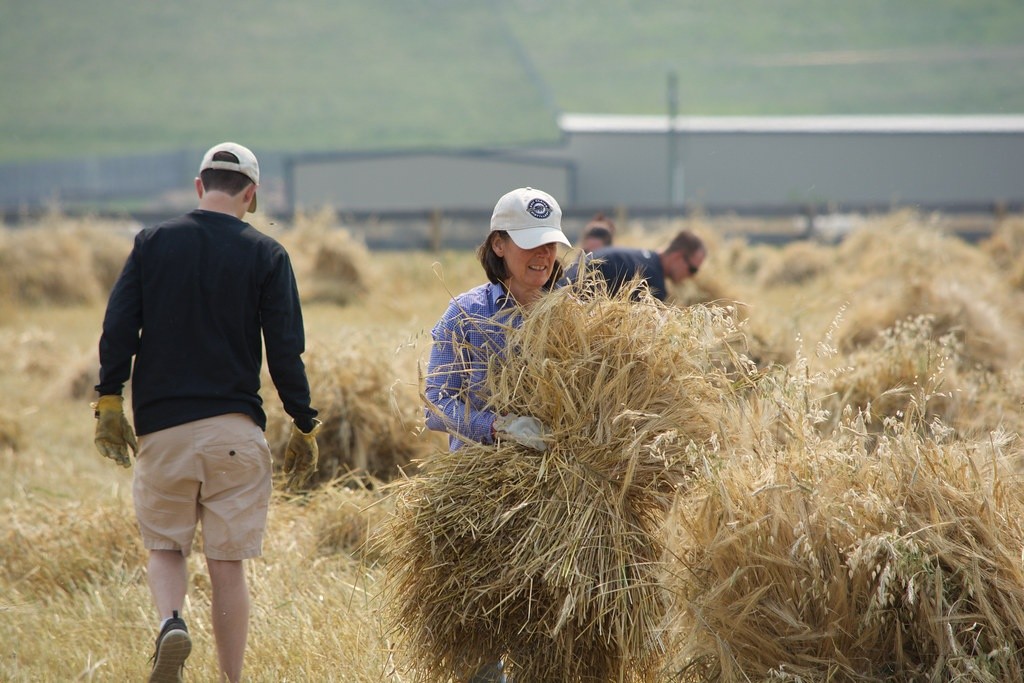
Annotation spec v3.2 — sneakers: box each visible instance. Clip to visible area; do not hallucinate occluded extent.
[146,611,192,682]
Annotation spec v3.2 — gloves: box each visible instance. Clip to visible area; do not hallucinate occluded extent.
[88,394,138,468]
[284,417,323,491]
[491,412,552,452]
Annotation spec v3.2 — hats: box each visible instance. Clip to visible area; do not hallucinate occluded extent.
[490,186,574,249]
[199,142,259,213]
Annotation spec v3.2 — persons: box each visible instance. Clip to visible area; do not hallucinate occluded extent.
[418,186,578,455]
[563,220,710,303]
[92,137,322,683]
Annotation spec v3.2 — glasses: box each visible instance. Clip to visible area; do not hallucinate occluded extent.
[686,258,699,275]
